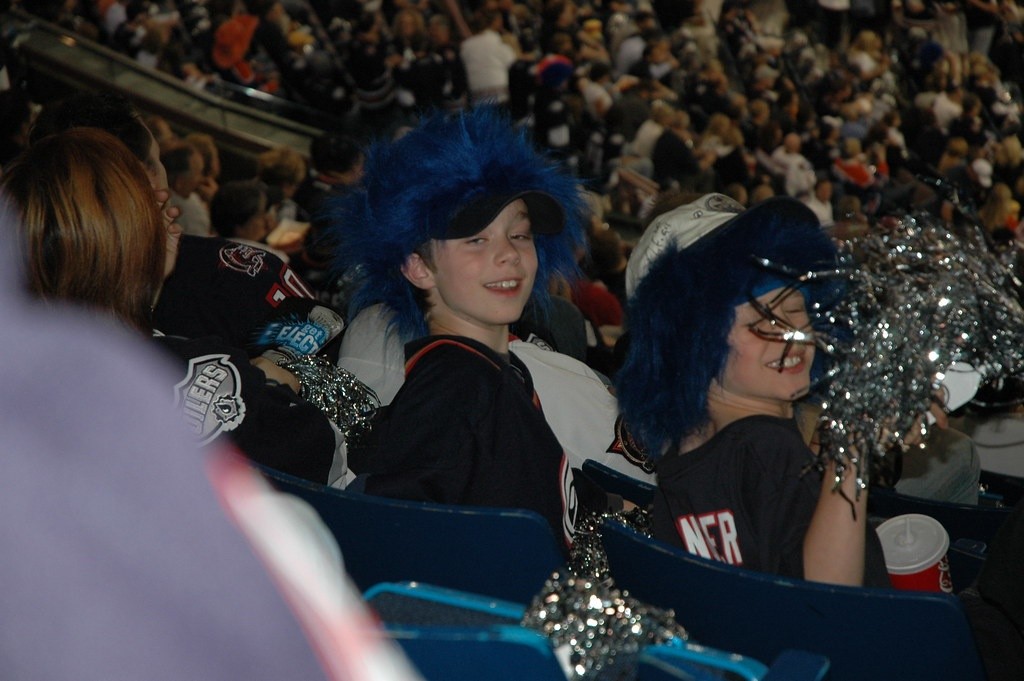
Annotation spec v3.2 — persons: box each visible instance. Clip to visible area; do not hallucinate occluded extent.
[0,0,1024,681]
[334,93,642,570]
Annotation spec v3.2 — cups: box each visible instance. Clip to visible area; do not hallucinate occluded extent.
[874,513,954,595]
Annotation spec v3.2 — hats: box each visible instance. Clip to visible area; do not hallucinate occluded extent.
[972,158,993,187]
[624,192,821,311]
[419,159,563,240]
[213,14,259,67]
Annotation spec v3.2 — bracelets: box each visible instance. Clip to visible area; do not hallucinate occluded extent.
[808,439,823,449]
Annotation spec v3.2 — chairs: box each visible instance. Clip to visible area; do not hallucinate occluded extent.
[246,453,1024,681]
